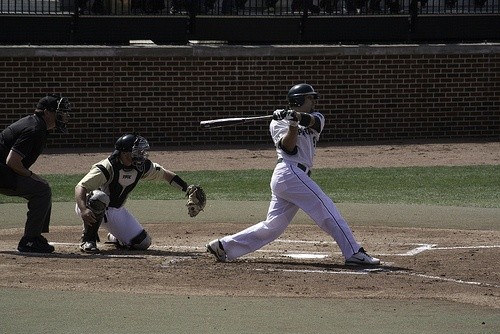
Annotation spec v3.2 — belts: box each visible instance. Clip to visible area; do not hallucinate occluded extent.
[277,158,311,177]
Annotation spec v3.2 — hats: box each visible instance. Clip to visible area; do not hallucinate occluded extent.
[37,96,60,112]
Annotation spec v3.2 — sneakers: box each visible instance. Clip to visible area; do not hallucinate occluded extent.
[345,247,380,265]
[206,239,226,263]
[17,235,54,253]
[79,240,97,253]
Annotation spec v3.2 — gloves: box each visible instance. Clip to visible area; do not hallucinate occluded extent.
[286,110,298,127]
[272,109,287,120]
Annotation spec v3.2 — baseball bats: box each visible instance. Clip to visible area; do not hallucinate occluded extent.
[199,109,296,129]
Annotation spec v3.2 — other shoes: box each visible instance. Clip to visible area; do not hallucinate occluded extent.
[107,233,112,241]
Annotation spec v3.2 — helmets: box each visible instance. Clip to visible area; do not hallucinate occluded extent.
[287,84,318,107]
[111,134,149,167]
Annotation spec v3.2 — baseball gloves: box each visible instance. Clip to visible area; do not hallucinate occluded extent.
[184,183,207,218]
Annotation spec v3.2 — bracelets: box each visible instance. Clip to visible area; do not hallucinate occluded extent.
[30,171,32,177]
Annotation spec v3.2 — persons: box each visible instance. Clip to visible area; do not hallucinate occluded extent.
[0,97,60,252]
[206,84,380,267]
[74,134,194,253]
